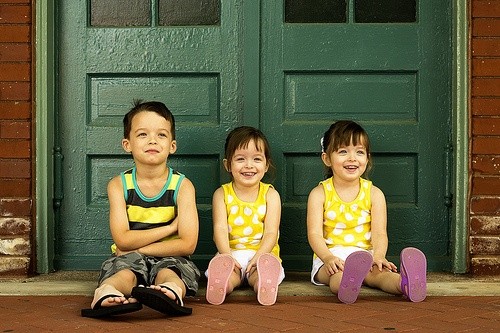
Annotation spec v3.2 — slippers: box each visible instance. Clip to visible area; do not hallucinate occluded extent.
[131,285,192,316]
[81,295,142,316]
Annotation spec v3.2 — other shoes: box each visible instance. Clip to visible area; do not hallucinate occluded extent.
[257,253,281,305]
[206,254,235,305]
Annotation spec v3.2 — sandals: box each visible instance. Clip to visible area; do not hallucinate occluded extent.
[337,250,373,304]
[399,248,426,302]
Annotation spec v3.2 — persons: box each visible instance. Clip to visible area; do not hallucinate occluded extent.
[205,126,285,306]
[81,99,199,314]
[306,120,427,305]
